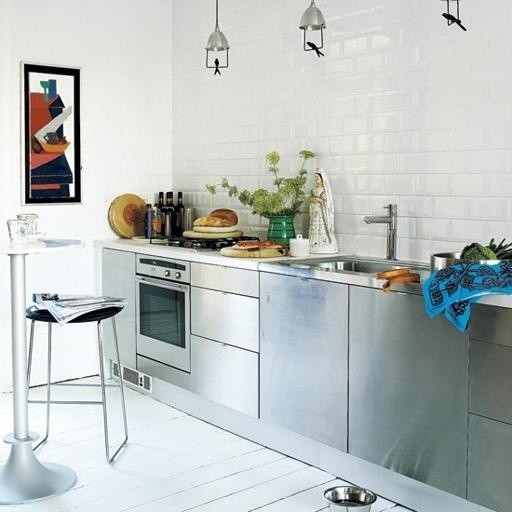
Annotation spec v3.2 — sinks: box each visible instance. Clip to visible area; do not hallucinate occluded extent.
[279,255,415,275]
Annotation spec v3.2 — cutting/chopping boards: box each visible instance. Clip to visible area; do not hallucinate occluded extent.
[219,245,288,258]
[182,225,244,239]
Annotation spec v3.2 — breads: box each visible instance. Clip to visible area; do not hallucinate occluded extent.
[209,209,238,225]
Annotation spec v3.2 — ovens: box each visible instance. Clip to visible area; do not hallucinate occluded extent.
[134,275,191,391]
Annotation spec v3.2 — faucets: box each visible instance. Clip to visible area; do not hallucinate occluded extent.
[362,203,399,260]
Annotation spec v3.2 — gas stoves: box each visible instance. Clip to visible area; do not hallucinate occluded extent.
[136,234,260,285]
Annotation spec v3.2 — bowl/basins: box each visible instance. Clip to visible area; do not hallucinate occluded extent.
[429,250,512,278]
[41,140,71,154]
[322,485,378,512]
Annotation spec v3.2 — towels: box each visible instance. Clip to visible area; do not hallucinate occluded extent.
[417,259,512,330]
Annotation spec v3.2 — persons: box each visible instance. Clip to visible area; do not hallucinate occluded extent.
[308,167,336,247]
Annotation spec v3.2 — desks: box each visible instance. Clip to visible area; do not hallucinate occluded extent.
[1,235,97,507]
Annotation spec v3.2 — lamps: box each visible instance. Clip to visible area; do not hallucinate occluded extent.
[201,1,232,78]
[296,1,326,56]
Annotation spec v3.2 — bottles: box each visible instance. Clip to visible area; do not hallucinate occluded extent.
[31,138,42,154]
[144,190,187,236]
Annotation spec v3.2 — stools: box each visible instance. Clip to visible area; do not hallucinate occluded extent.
[23,297,131,463]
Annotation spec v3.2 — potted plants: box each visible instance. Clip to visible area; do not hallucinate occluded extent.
[201,147,319,254]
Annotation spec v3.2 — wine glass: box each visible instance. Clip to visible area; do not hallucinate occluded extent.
[40,77,50,96]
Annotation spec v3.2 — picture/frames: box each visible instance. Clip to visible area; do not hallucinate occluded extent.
[19,58,85,208]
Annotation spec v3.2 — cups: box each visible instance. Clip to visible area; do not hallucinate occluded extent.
[47,78,56,99]
[184,208,195,231]
[288,237,311,258]
[15,213,40,240]
[6,219,30,248]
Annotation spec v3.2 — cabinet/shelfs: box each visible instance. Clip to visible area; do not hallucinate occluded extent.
[187,259,263,443]
[255,267,348,482]
[346,273,469,511]
[99,245,136,374]
[461,299,512,512]
